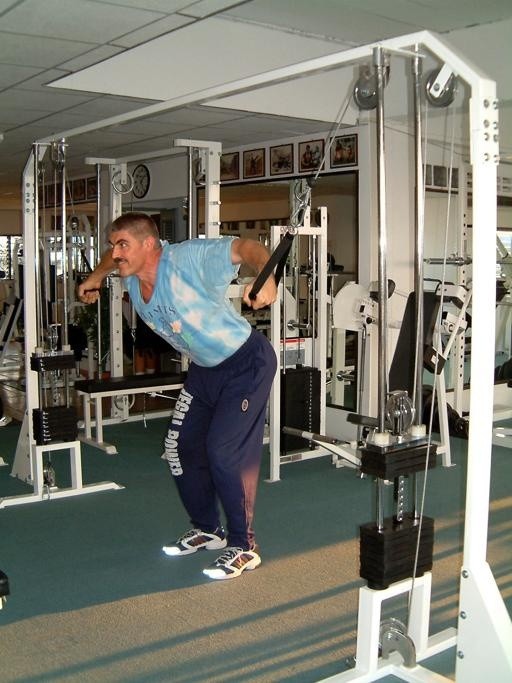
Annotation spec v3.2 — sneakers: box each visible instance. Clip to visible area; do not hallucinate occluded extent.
[161,525,228,557]
[201,544,263,580]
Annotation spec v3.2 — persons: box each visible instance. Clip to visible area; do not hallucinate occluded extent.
[249,152,259,175]
[302,145,313,166]
[76,211,278,581]
[348,144,353,161]
[311,146,321,166]
[335,140,344,162]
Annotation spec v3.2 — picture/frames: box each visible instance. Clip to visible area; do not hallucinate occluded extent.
[220,133,358,181]
[38,175,98,209]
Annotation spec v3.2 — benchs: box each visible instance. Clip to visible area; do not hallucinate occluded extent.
[73,372,188,456]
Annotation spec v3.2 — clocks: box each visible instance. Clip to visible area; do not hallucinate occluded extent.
[131,164,150,199]
